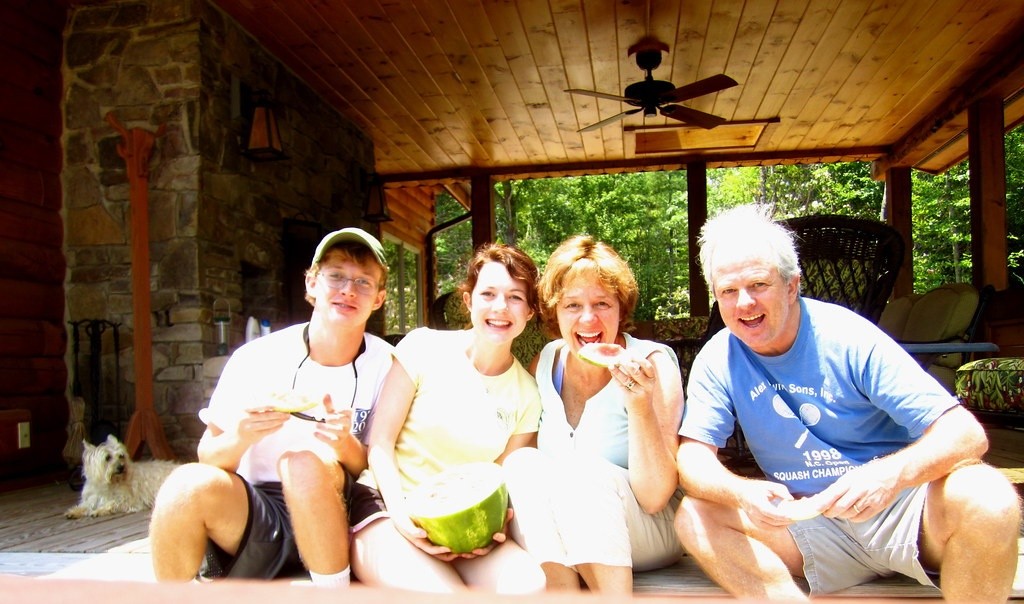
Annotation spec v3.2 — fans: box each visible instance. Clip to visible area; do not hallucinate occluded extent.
[563,42,739,132]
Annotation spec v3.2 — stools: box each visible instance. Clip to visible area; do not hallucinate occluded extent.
[955,357,1024,428]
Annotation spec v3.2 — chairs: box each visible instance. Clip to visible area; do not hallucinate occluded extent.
[432,215,1000,468]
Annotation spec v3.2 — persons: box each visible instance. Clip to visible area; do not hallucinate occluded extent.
[503,236,683,600]
[349,244,543,594]
[674,208,1021,604]
[149,227,394,586]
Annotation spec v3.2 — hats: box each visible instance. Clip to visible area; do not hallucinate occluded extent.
[311,228,387,270]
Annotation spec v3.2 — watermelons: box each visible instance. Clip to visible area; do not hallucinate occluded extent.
[408,477,508,554]
[575,343,633,367]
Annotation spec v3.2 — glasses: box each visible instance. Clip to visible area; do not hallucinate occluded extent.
[290,353,358,423]
[317,272,380,295]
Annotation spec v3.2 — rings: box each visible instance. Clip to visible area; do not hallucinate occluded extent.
[853,505,860,514]
[627,380,636,389]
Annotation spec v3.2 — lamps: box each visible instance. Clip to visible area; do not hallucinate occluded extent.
[360,168,394,224]
[232,75,283,162]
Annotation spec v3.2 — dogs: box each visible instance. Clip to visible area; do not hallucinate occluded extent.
[63,434,182,520]
